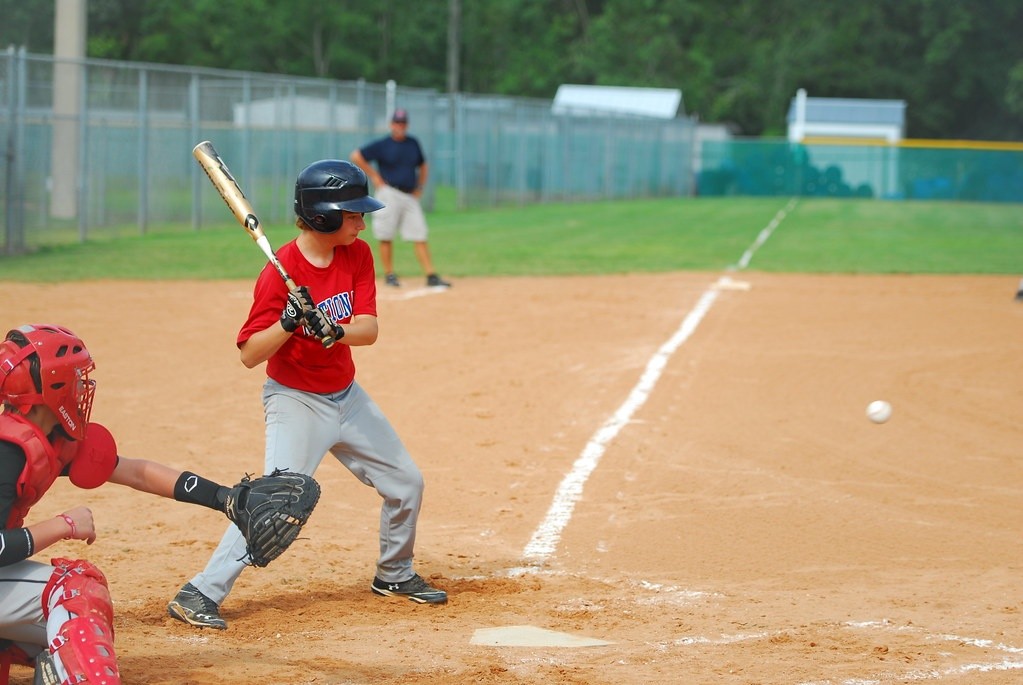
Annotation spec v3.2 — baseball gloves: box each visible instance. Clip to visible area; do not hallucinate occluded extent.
[222,467,321,568]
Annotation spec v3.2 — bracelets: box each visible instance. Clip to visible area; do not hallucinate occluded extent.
[56,514,77,540]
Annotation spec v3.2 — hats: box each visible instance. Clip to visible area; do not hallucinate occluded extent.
[393,108,407,123]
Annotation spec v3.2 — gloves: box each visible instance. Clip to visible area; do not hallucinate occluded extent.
[280,286,315,332]
[301,308,345,349]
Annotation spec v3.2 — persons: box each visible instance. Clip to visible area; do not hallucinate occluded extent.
[0,322,321,685]
[168,158,448,630]
[350,107,453,289]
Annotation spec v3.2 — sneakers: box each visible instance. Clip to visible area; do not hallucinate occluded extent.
[371,573,447,603]
[167,582,227,630]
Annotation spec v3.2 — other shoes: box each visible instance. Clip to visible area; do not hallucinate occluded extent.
[428,275,451,286]
[386,274,398,286]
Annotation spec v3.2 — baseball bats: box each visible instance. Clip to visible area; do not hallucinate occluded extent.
[192,138,336,351]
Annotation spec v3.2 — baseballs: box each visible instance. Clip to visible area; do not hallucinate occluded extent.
[866,399,892,425]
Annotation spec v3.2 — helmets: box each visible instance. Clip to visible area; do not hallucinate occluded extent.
[294,159,385,232]
[0,323,96,441]
